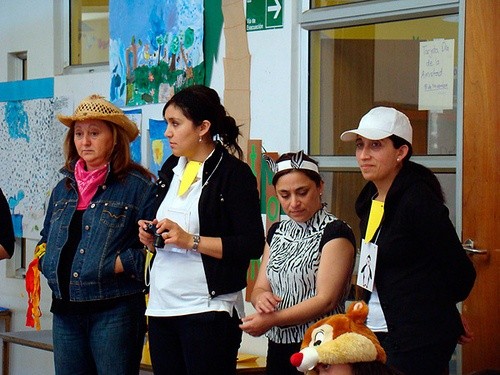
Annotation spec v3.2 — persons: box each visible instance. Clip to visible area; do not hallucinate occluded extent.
[0,188,16,260]
[239,150,357,375]
[290,301,387,375]
[134,86,265,375]
[35,94,154,375]
[340,106,476,373]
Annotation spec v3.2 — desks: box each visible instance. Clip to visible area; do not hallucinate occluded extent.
[0,309,16,375]
[0,330,267,375]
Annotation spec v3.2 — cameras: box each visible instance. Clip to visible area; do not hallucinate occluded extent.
[143,223,168,248]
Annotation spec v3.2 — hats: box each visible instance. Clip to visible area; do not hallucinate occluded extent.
[340,107,412,144]
[57,94,138,142]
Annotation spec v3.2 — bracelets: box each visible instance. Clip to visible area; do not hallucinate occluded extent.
[192,235,200,253]
